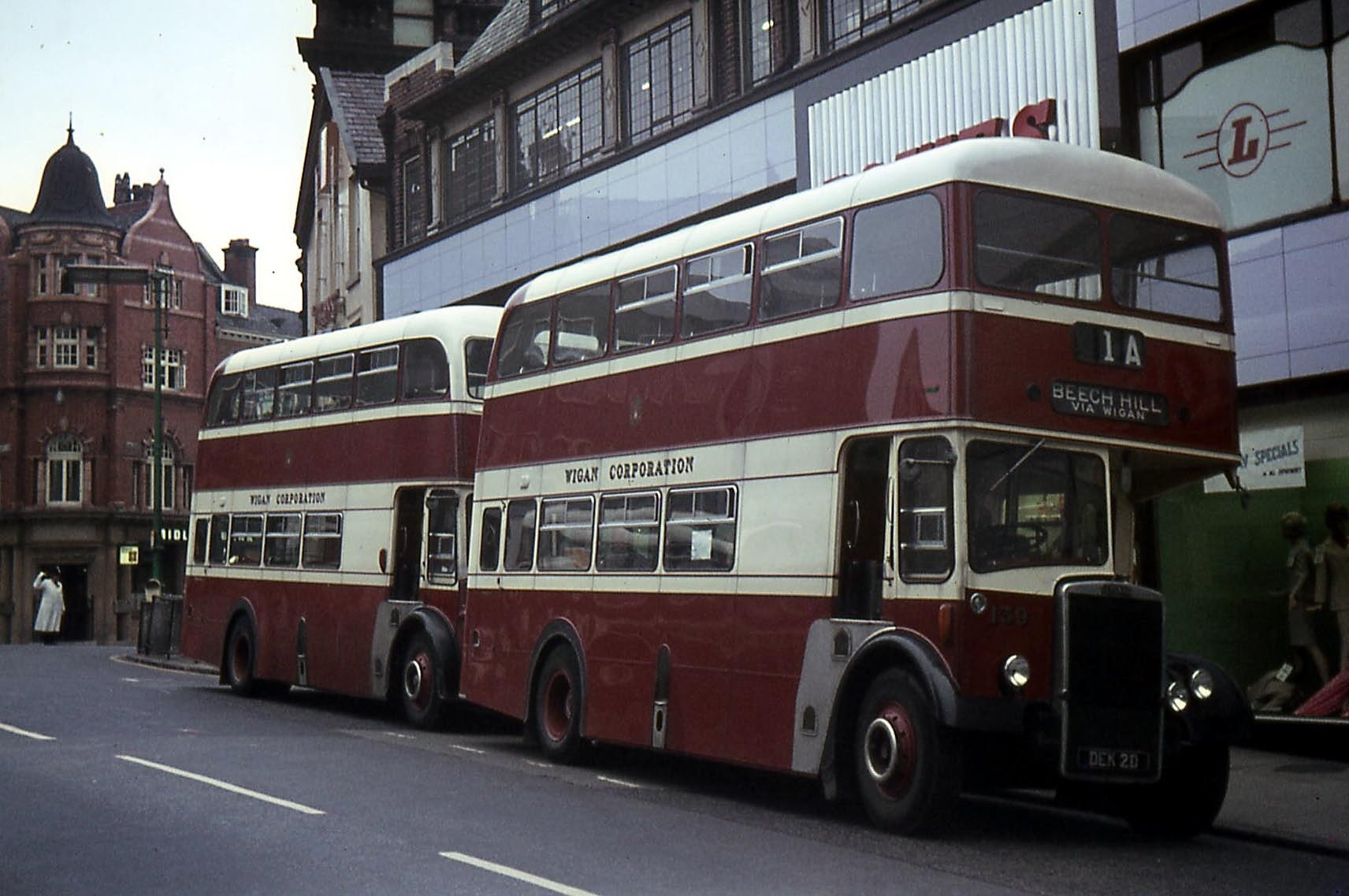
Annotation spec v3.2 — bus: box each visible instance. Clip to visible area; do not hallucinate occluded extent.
[178,300,604,737]
[461,133,1244,836]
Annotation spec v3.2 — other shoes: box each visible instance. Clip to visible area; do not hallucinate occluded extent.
[44,640,57,646]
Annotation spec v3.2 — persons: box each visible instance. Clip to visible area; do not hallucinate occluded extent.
[31,564,68,647]
[1244,666,1295,711]
[1306,502,1348,719]
[1264,511,1333,692]
[601,527,652,569]
[967,506,1015,567]
[1112,276,1136,310]
[523,347,546,374]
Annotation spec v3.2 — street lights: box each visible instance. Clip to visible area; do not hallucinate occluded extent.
[63,259,169,602]
